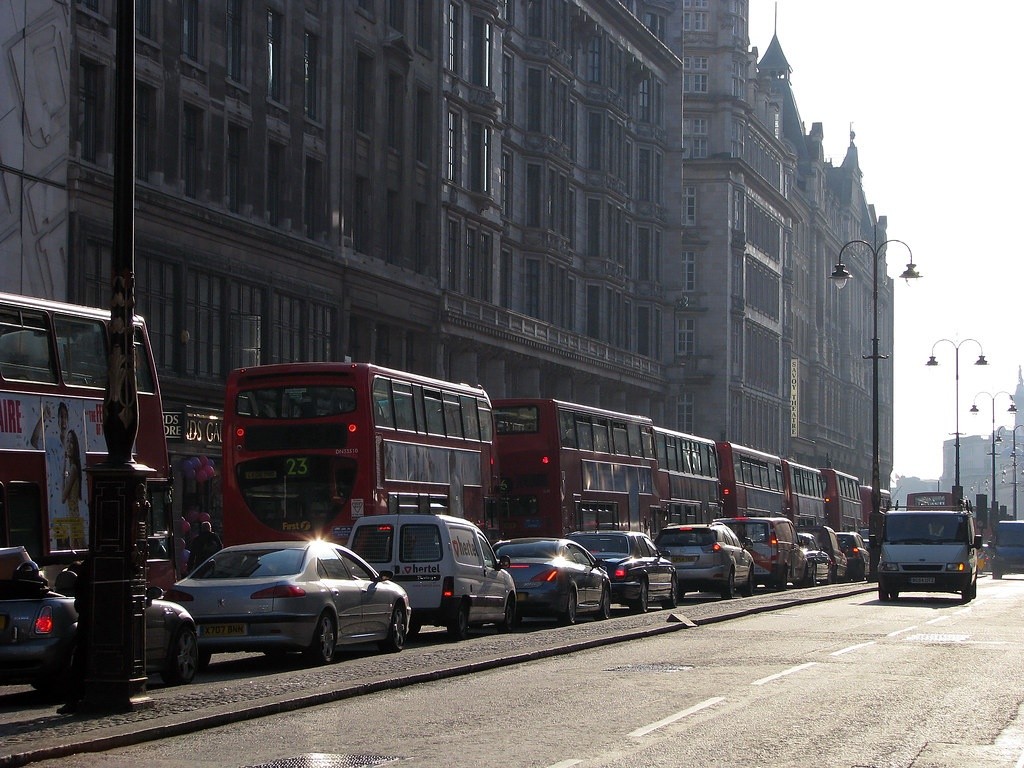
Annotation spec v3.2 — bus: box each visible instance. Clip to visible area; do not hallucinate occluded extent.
[648,427,728,535]
[780,459,830,527]
[905,491,970,511]
[857,485,893,527]
[0,293,179,566]
[492,395,655,537]
[821,468,865,530]
[715,439,790,520]
[219,359,501,550]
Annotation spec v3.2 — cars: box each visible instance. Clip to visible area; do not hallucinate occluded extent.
[484,537,612,627]
[0,561,200,701]
[794,532,833,587]
[796,525,850,583]
[836,531,874,582]
[166,539,414,668]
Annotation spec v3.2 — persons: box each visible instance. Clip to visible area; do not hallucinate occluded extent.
[31,402,87,550]
[449,451,463,518]
[187,521,223,570]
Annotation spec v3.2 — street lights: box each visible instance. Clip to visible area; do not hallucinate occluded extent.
[828,239,924,580]
[970,392,1024,550]
[925,338,989,512]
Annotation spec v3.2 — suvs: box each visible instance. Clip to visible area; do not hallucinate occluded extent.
[566,530,678,613]
[714,516,811,591]
[653,521,757,598]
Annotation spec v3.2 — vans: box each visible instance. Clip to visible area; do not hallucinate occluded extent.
[877,508,983,603]
[988,521,1023,580]
[345,514,520,636]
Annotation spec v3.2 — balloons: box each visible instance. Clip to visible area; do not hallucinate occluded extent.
[181,511,210,545]
[183,456,216,482]
[181,538,190,574]
